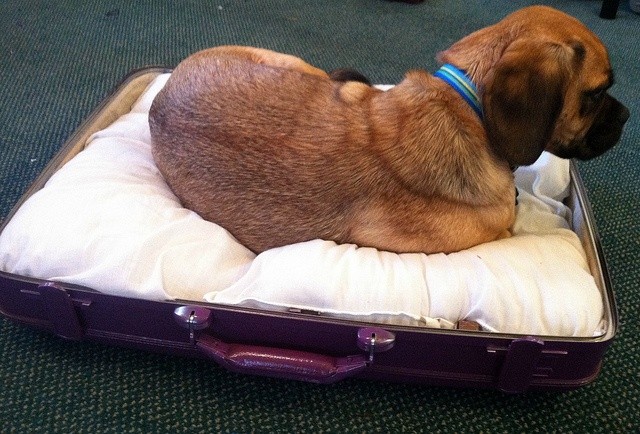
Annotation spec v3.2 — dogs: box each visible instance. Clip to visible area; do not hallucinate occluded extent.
[149,6,631,256]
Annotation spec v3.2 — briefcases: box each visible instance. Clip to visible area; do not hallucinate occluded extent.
[0,67,619,395]
